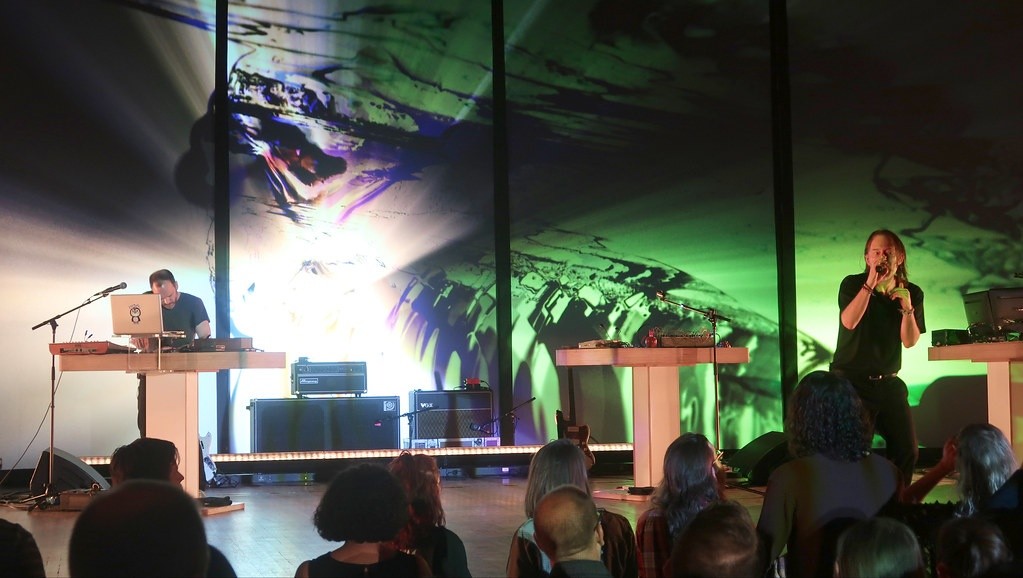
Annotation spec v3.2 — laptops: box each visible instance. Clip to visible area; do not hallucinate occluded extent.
[962,287,1023,334]
[110,295,185,335]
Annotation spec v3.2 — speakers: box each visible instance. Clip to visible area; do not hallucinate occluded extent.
[250,397,401,452]
[30,447,111,496]
[727,431,793,486]
[409,388,496,438]
[290,362,366,393]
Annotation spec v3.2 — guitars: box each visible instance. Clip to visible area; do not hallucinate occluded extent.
[199,432,217,481]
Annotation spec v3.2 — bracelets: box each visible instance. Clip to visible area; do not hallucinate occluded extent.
[900,308,914,314]
[863,283,873,293]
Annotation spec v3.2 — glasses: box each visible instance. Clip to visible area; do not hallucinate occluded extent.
[594,510,604,532]
[712,450,723,464]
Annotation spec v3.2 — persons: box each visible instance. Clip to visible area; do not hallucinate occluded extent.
[108,438,237,578]
[830,230,926,487]
[130,269,212,491]
[532,484,613,578]
[663,499,759,578]
[834,422,1023,578]
[385,453,473,578]
[635,432,759,578]
[294,464,433,578]
[754,371,906,578]
[506,439,637,578]
[67,480,208,578]
[0,518,45,578]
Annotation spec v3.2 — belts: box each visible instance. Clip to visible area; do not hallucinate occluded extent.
[831,368,897,381]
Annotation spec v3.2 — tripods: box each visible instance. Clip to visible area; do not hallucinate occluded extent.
[19,292,110,511]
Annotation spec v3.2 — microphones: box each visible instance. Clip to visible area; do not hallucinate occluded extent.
[375,417,392,426]
[876,264,885,273]
[94,283,127,296]
[470,423,491,435]
